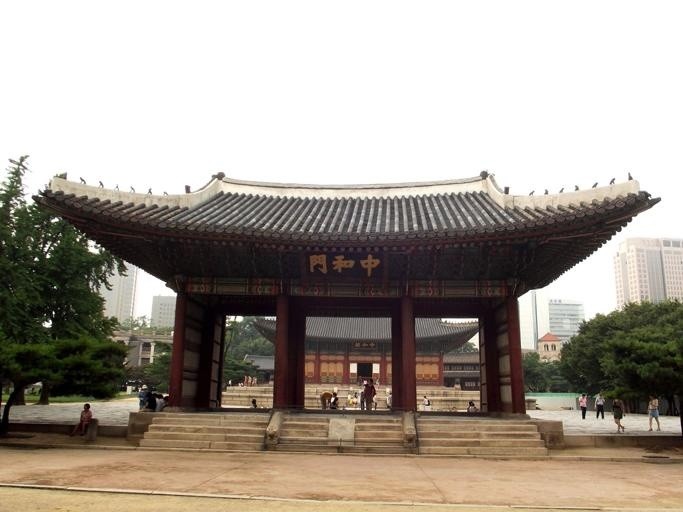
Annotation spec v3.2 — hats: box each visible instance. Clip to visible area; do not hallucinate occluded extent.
[142,385,148,390]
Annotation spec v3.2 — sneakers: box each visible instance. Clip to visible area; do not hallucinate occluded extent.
[617,426,625,434]
[649,427,661,431]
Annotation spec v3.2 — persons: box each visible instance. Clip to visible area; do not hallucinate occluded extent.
[238,374,257,388]
[593,392,606,420]
[136,385,169,412]
[68,403,92,436]
[611,398,625,432]
[249,399,257,409]
[577,393,587,419]
[421,395,430,406]
[466,401,478,412]
[316,377,391,410]
[646,393,660,433]
